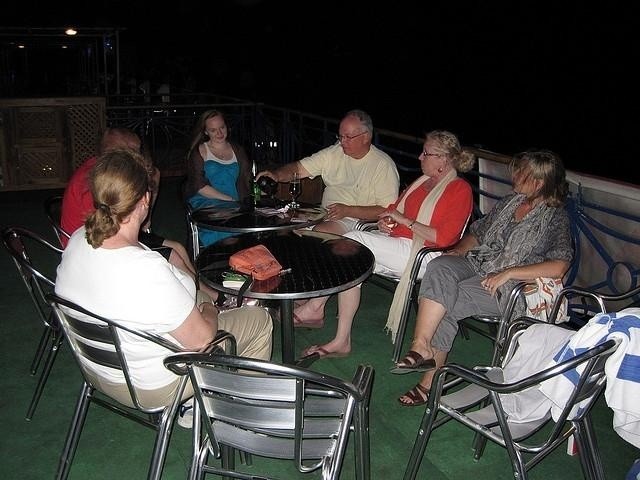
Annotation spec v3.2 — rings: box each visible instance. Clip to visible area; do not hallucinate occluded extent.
[333,215,336,218]
[485,284,489,288]
[492,287,495,290]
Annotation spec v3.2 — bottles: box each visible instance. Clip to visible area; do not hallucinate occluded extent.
[250,160,260,206]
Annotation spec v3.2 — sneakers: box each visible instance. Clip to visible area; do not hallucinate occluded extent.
[177,400,194,429]
[222,293,259,308]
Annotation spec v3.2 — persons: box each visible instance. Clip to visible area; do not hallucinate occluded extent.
[256,111,401,323]
[292,129,477,359]
[189,109,252,250]
[391,149,573,405]
[55,148,273,438]
[63,125,259,314]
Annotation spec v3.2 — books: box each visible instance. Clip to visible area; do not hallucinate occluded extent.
[223,268,253,289]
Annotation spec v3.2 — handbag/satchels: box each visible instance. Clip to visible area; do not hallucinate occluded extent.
[228,244,283,281]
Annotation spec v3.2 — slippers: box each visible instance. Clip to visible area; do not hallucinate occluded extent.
[303,344,347,359]
[390,351,436,375]
[396,383,447,406]
[276,310,325,329]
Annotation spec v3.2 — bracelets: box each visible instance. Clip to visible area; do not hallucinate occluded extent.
[408,220,416,232]
[230,196,235,202]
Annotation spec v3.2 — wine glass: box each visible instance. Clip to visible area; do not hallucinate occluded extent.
[287,171,301,209]
[386,217,395,233]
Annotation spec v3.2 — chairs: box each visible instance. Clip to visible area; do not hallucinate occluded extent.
[42,195,71,346]
[394,212,580,367]
[45,293,252,480]
[336,211,474,339]
[163,351,376,480]
[181,178,210,261]
[403,285,623,480]
[497,285,640,368]
[2,223,64,421]
[313,166,408,230]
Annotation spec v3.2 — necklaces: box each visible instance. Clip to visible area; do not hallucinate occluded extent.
[209,141,228,159]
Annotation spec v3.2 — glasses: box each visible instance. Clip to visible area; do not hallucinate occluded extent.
[423,150,445,157]
[335,131,370,140]
